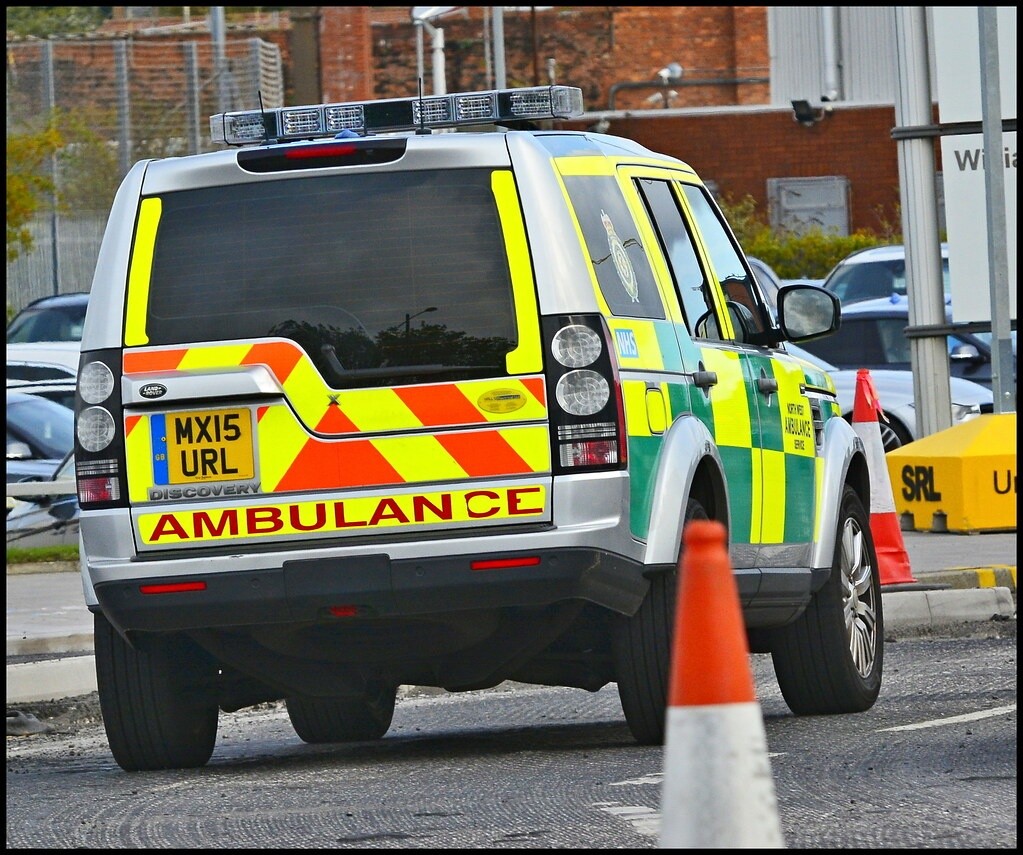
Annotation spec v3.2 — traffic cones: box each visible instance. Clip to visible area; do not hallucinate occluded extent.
[851,369,917,585]
[659,521,787,849]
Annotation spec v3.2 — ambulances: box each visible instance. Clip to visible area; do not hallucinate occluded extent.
[76,85,884,772]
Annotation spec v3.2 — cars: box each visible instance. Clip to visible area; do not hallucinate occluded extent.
[6,292,90,343]
[786,294,1017,416]
[782,339,994,453]
[822,242,951,307]
[6,342,83,412]
[6,391,76,517]
[748,256,785,319]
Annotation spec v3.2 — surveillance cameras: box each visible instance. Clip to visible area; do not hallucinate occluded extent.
[820,89,838,111]
[658,67,671,86]
